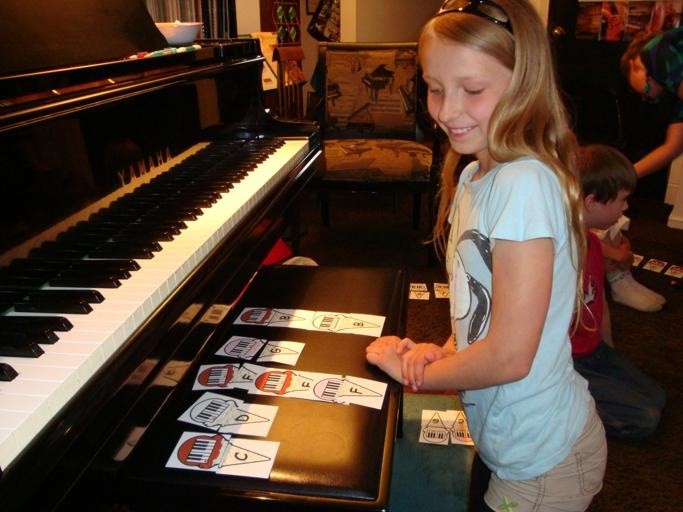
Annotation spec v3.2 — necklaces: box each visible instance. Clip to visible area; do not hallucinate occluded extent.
[641,72,667,105]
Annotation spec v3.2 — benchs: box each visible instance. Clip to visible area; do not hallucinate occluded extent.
[107,263,417,511]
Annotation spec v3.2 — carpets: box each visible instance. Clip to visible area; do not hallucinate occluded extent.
[381,384,477,511]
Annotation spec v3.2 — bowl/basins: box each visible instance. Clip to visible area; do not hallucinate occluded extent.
[154,21,202,46]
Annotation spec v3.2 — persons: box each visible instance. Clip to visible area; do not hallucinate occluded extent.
[569,144,668,439]
[365,0,609,512]
[590,215,666,312]
[619,29,683,179]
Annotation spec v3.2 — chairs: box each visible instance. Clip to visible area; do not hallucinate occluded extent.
[315,40,433,232]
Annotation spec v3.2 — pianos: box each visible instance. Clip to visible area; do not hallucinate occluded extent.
[0,0,324,512]
